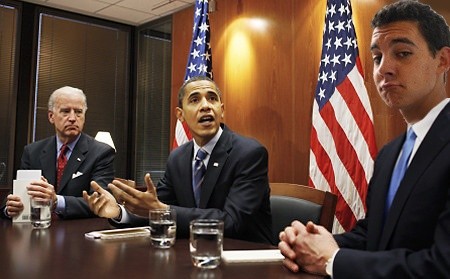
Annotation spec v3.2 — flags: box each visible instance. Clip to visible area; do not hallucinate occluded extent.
[308,1,378,234]
[171,0,216,151]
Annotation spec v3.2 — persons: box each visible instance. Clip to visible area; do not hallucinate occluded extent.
[278,0,449,279]
[0,85,118,221]
[82,75,273,245]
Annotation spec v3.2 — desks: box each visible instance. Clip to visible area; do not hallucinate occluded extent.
[0,219,324,279]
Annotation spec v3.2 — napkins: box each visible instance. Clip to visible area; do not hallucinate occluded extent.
[222,249,284,263]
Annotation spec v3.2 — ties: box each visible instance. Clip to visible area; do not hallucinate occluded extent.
[56,144,71,191]
[193,150,208,209]
[387,127,417,213]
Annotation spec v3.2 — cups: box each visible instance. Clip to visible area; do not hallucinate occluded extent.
[149,209,177,249]
[190,219,224,269]
[30,197,51,229]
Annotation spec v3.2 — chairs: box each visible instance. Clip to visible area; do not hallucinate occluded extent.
[261,183,338,248]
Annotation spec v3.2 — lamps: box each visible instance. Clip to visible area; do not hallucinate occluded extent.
[94,131,116,151]
